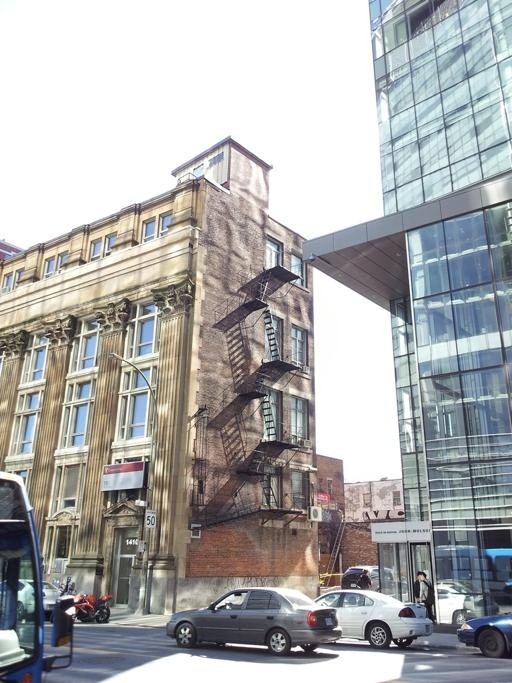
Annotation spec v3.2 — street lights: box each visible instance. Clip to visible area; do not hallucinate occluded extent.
[109,353,157,617]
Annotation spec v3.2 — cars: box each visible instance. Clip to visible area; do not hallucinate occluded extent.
[17,579,63,620]
[167,587,433,657]
[341,567,406,589]
[457,612,512,658]
[432,580,499,624]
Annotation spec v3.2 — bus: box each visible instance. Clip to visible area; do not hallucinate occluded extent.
[434,545,512,600]
[0,471,73,683]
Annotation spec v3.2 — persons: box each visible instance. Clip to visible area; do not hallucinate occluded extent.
[418,572,434,621]
[413,572,422,603]
[356,570,371,589]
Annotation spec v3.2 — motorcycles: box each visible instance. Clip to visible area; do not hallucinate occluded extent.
[50,594,112,624]
